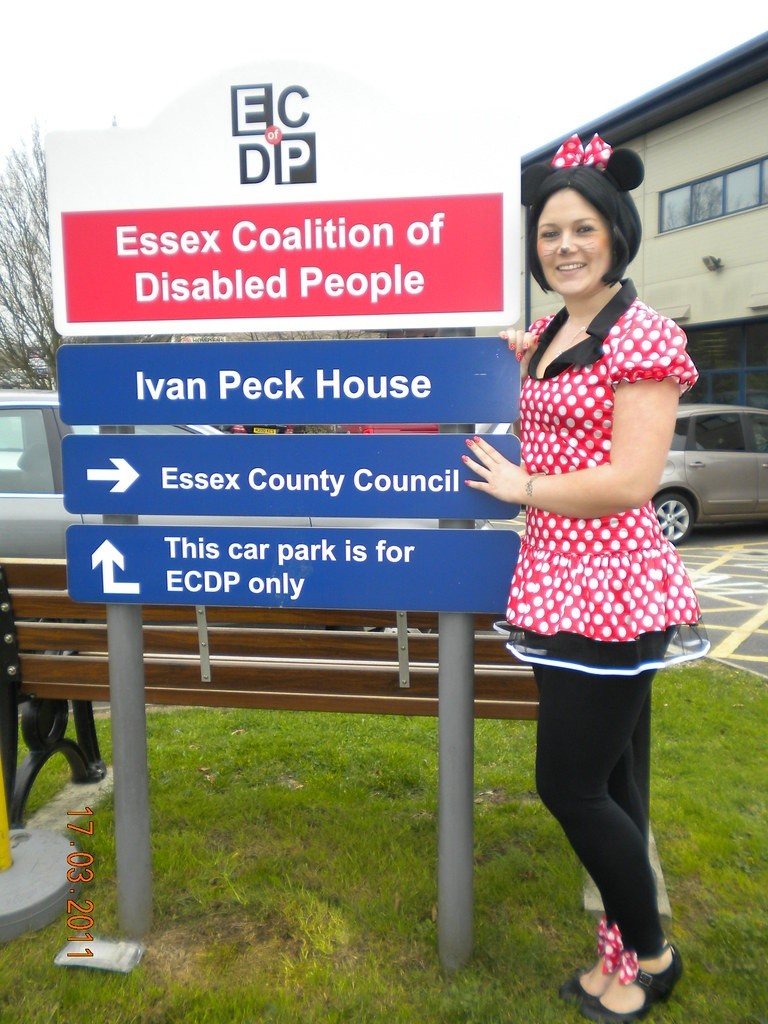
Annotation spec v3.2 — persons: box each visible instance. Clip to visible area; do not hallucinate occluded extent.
[461,134,710,1024]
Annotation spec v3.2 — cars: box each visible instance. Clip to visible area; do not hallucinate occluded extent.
[0,385,229,596]
[509,402,767,544]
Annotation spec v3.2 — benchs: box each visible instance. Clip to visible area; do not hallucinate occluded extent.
[1,557,540,826]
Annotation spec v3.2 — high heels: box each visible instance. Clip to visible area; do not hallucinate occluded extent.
[559,916,624,1001]
[578,924,682,1024]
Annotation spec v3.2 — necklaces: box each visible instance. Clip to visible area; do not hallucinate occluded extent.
[556,326,587,357]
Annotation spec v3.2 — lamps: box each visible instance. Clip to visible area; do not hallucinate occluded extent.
[702,256,725,271]
